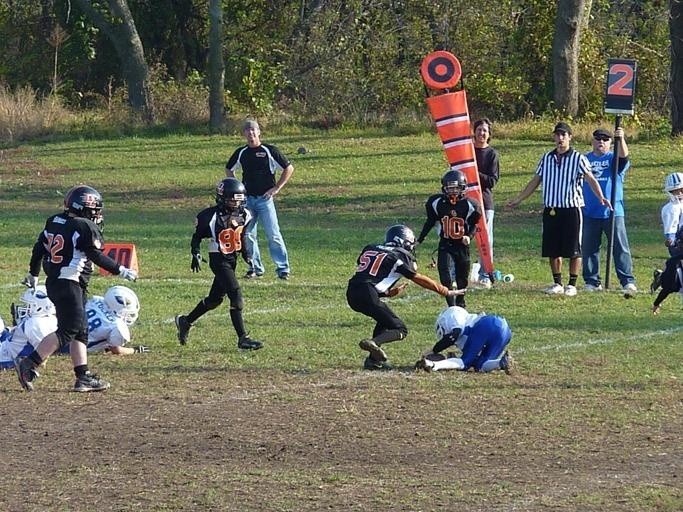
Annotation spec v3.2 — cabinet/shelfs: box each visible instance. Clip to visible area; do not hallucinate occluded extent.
[494,271,502,281]
[502,274,515,283]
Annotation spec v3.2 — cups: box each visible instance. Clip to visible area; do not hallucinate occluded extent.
[595,137,610,142]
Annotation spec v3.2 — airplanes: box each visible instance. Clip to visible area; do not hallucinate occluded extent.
[388,282,407,297]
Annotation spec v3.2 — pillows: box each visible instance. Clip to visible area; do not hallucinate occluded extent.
[385,224,417,251]
[441,170,467,200]
[438,306,469,336]
[215,178,247,207]
[23,284,57,318]
[103,285,140,322]
[664,172,683,201]
[63,184,104,219]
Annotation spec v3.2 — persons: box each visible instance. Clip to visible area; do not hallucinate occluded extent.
[650,171,683,314]
[412,170,482,305]
[174,176,262,350]
[422,306,511,373]
[464,118,499,286]
[1,185,149,392]
[225,120,295,278]
[507,121,614,296]
[581,127,638,294]
[346,223,467,370]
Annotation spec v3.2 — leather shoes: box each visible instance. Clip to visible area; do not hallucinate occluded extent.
[21,273,39,296]
[118,264,139,283]
[132,344,151,354]
[190,251,208,273]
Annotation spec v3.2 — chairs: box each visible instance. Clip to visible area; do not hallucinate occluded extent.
[414,357,434,371]
[499,353,514,375]
[73,370,111,392]
[363,357,392,370]
[478,277,492,290]
[14,357,40,391]
[564,285,577,296]
[175,314,194,346]
[584,283,603,291]
[650,269,664,295]
[544,282,565,295]
[358,339,388,362]
[256,273,264,279]
[623,282,637,291]
[238,334,265,351]
[279,272,290,280]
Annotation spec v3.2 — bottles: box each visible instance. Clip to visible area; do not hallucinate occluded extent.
[592,129,613,139]
[552,123,572,137]
